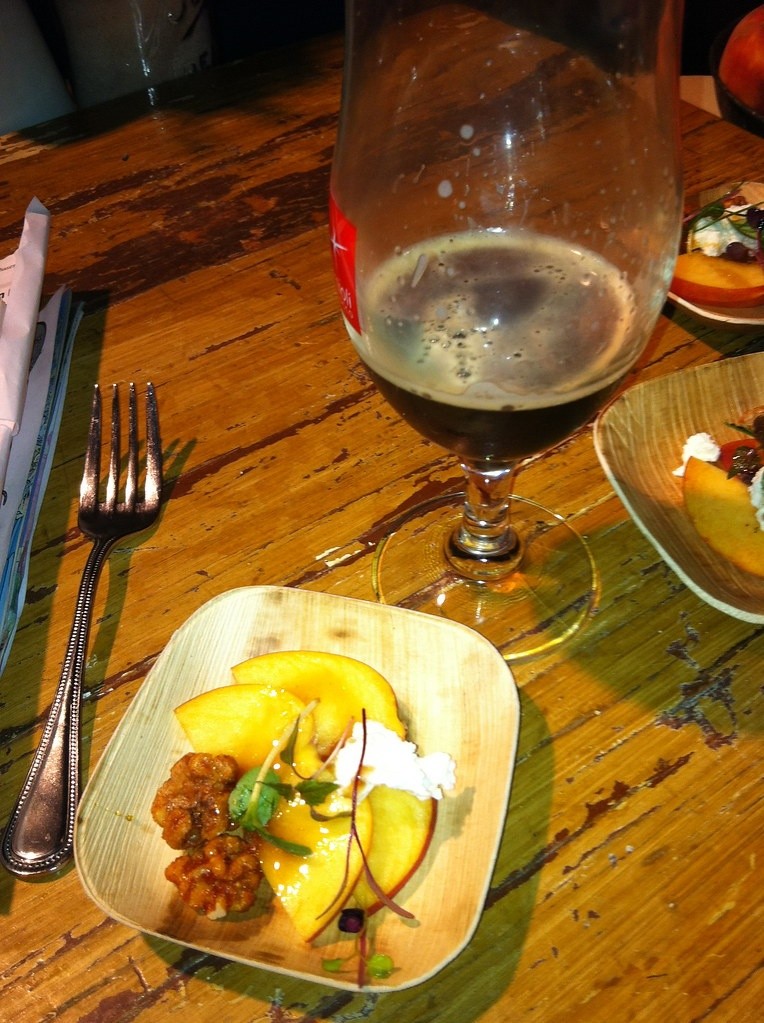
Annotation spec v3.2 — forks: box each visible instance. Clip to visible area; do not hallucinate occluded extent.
[1,380,164,879]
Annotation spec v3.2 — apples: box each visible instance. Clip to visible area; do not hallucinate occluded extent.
[174,647,437,942]
[669,243,764,309]
[682,457,764,577]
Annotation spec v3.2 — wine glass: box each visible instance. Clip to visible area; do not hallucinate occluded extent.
[329,1,682,660]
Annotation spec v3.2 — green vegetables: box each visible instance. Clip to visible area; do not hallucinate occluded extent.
[688,179,764,244]
[217,698,416,988]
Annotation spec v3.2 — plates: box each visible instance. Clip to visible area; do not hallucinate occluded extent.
[667,182,764,332]
[74,587,521,992]
[593,350,764,624]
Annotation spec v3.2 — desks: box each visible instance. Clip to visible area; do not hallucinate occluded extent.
[0,0,763,1023]
